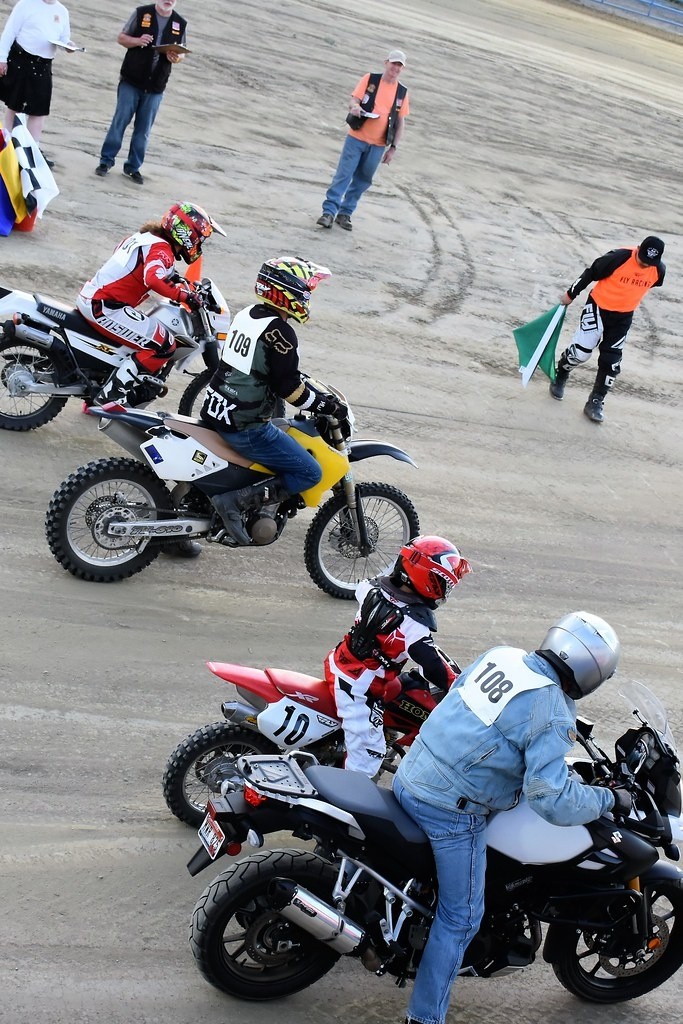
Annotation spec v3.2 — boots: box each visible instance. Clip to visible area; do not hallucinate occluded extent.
[212,476,289,546]
[585,368,617,422]
[94,352,153,406]
[549,347,585,400]
[83,402,98,416]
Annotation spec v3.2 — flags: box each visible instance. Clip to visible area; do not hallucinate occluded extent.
[513,303,567,385]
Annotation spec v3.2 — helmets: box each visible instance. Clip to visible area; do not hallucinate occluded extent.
[255,257,331,324]
[162,202,226,265]
[539,611,620,698]
[394,536,461,609]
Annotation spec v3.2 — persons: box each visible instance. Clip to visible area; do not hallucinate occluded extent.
[312,535,470,863]
[0,0,76,168]
[95,0,187,183]
[391,612,631,1024]
[157,258,332,557]
[317,50,410,230]
[550,236,666,422]
[77,201,226,417]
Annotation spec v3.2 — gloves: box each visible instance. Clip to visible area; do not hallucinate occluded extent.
[608,787,632,817]
[333,400,348,420]
[170,270,180,282]
[186,292,203,310]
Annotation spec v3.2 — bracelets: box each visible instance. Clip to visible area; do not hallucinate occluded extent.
[391,145,396,148]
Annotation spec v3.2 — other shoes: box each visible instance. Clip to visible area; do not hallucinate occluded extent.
[123,170,143,182]
[39,147,55,168]
[95,164,108,175]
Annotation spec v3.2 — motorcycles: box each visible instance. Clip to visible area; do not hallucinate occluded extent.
[185,710,683,1005]
[0,270,231,435]
[162,642,463,829]
[44,356,421,601]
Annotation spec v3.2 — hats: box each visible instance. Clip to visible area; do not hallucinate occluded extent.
[387,50,405,67]
[638,236,665,266]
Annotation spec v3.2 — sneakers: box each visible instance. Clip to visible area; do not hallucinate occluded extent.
[335,214,352,230]
[317,213,333,228]
